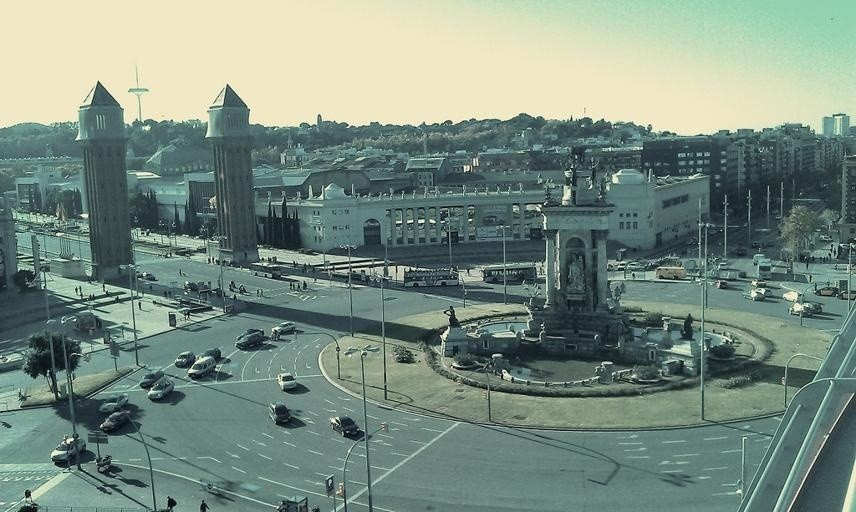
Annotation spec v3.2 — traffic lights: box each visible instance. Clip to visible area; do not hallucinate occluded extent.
[337,483,343,496]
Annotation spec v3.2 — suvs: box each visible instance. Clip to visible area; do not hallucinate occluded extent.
[268,402,292,425]
[185,281,198,291]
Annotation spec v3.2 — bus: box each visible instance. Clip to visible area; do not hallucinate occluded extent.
[404,264,459,288]
[479,263,538,284]
[656,266,687,280]
[250,262,282,280]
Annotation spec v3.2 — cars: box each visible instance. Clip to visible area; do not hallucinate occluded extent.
[99,392,129,414]
[139,369,164,388]
[329,415,360,437]
[50,437,86,464]
[277,373,297,391]
[99,410,132,433]
[175,351,195,367]
[136,271,155,281]
[148,376,176,401]
[708,209,856,318]
[235,320,296,350]
[606,256,661,272]
[198,347,221,362]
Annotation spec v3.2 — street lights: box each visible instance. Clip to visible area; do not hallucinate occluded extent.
[448,268,466,308]
[120,262,143,365]
[459,366,491,424]
[133,215,207,254]
[210,235,229,303]
[68,352,91,393]
[46,315,81,435]
[344,344,381,511]
[369,271,393,400]
[495,223,511,304]
[340,243,360,338]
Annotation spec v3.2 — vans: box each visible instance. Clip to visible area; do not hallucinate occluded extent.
[188,356,217,379]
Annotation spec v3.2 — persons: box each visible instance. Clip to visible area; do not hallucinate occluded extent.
[444,305,461,327]
[199,499,209,511]
[24,488,33,503]
[76,238,546,331]
[166,495,174,511]
[276,498,291,511]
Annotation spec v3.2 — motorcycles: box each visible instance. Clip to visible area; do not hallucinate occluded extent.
[95,454,112,471]
